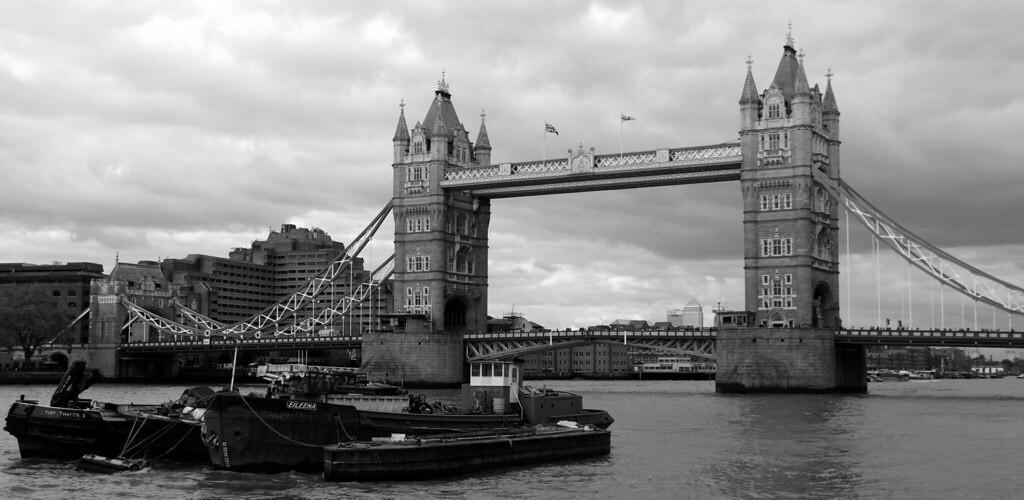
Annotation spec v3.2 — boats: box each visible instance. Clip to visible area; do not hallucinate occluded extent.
[5,359,612,482]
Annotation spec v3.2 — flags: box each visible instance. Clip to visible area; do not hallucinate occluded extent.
[545,123,560,136]
[621,114,636,123]
